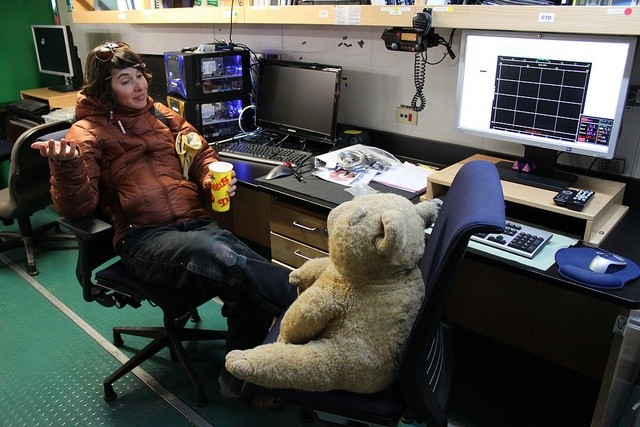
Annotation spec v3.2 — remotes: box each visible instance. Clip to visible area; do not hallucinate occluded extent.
[553,188,575,208]
[571,188,594,209]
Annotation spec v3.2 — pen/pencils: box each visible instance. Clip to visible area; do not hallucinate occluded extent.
[290,167,304,183]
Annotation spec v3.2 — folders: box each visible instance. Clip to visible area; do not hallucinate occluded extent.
[314,144,365,170]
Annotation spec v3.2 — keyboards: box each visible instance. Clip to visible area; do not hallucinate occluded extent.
[218,143,313,167]
[7,99,48,112]
[470,216,555,257]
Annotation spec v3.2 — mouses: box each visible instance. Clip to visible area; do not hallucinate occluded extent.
[265,165,294,179]
[589,250,629,273]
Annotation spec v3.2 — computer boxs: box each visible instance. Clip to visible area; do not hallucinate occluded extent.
[166,96,253,145]
[164,51,252,103]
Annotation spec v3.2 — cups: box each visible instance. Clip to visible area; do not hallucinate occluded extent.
[208,161,233,213]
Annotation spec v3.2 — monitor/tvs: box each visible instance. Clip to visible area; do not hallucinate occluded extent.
[30,24,74,92]
[453,30,638,192]
[256,59,342,147]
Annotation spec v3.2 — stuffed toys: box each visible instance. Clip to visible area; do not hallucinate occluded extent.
[225,192,439,396]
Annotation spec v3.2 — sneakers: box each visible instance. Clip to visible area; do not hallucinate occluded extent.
[218,375,284,409]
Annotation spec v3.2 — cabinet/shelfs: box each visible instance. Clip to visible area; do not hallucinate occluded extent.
[19,83,81,109]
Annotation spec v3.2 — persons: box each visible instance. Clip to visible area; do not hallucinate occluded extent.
[30,38,305,410]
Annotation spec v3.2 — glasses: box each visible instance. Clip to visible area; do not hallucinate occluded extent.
[95,41,129,61]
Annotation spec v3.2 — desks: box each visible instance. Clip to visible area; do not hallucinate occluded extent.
[253,143,639,426]
[210,134,446,270]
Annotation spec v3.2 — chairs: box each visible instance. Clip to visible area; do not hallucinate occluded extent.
[239,159,506,426]
[0,119,72,275]
[55,210,228,408]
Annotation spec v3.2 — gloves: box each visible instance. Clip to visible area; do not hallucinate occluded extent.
[175,132,203,181]
